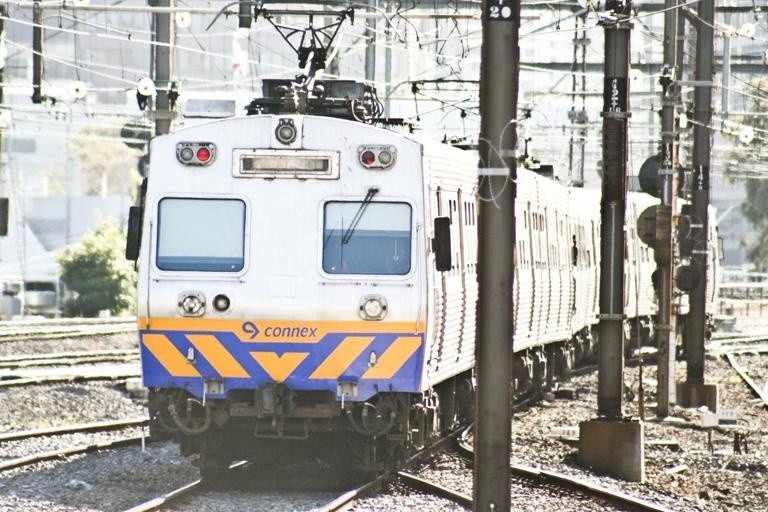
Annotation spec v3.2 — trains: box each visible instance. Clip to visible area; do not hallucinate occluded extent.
[122,69,718,481]
